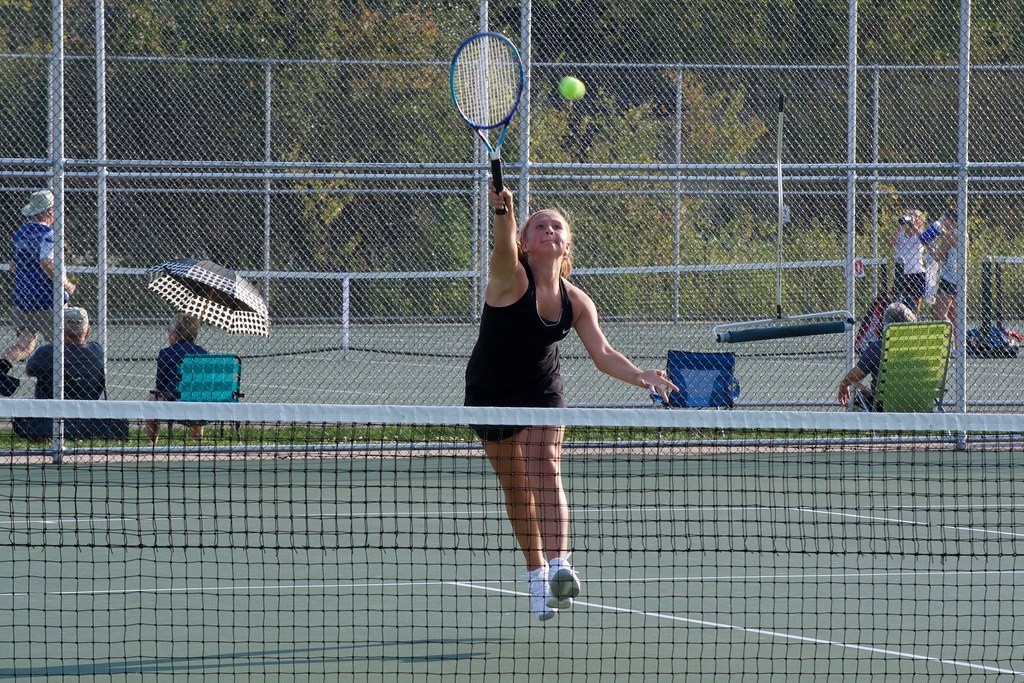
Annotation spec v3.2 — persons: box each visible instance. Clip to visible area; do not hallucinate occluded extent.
[0,190,76,375]
[838,302,920,411]
[26,306,105,400]
[464,177,679,620]
[146,314,209,443]
[888,207,971,359]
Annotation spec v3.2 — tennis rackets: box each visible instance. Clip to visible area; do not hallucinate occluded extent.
[450,32,526,216]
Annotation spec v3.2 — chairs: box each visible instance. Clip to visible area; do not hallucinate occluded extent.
[648,349,740,407]
[14,369,129,441]
[855,320,953,435]
[151,353,242,443]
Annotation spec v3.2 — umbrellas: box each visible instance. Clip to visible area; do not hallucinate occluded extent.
[144,258,269,338]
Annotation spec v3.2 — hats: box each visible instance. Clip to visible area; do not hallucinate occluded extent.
[21,189,55,216]
[63,306,89,334]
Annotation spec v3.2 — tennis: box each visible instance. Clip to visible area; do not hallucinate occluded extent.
[558,76,584,99]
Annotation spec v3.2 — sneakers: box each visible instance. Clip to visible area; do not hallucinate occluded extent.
[526,573,557,623]
[547,567,581,610]
